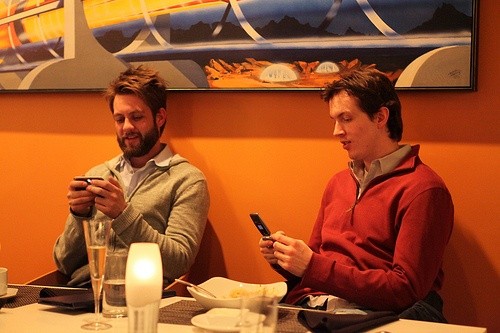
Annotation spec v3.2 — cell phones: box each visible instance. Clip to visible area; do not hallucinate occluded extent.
[74,176,104,190]
[250,213,282,270]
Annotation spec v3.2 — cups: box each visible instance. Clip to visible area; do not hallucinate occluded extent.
[0,267,8,295]
[125,242,163,333]
[238,292,277,333]
[102,250,129,317]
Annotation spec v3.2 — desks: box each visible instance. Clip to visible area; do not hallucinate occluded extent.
[0,284,487,333]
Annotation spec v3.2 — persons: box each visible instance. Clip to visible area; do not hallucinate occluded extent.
[54,66,210,290]
[258,63,455,324]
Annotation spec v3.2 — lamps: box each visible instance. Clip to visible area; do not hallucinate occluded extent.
[125,242,162,333]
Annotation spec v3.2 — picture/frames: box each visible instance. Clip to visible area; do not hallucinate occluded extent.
[0,0,479,94]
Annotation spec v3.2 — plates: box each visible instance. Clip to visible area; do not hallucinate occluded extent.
[0,288,19,299]
[191,306,266,332]
[186,277,288,313]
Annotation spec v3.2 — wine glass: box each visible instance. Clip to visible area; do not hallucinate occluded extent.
[82,217,114,331]
